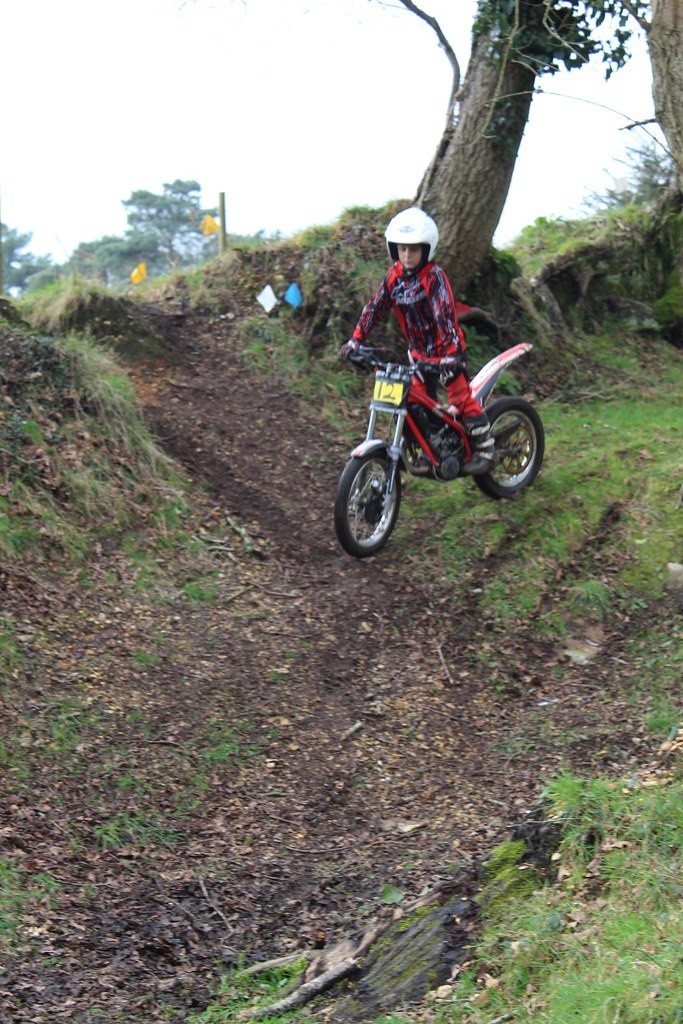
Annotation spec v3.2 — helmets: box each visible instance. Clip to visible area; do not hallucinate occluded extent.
[384,207,438,262]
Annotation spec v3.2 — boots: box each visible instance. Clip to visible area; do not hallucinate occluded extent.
[411,454,435,479]
[461,413,500,473]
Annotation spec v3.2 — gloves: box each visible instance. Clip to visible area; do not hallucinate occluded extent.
[437,356,459,374]
[340,338,359,360]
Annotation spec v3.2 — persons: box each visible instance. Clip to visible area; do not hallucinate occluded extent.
[338,206,498,450]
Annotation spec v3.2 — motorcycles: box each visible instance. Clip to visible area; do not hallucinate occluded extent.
[326,341,544,559]
